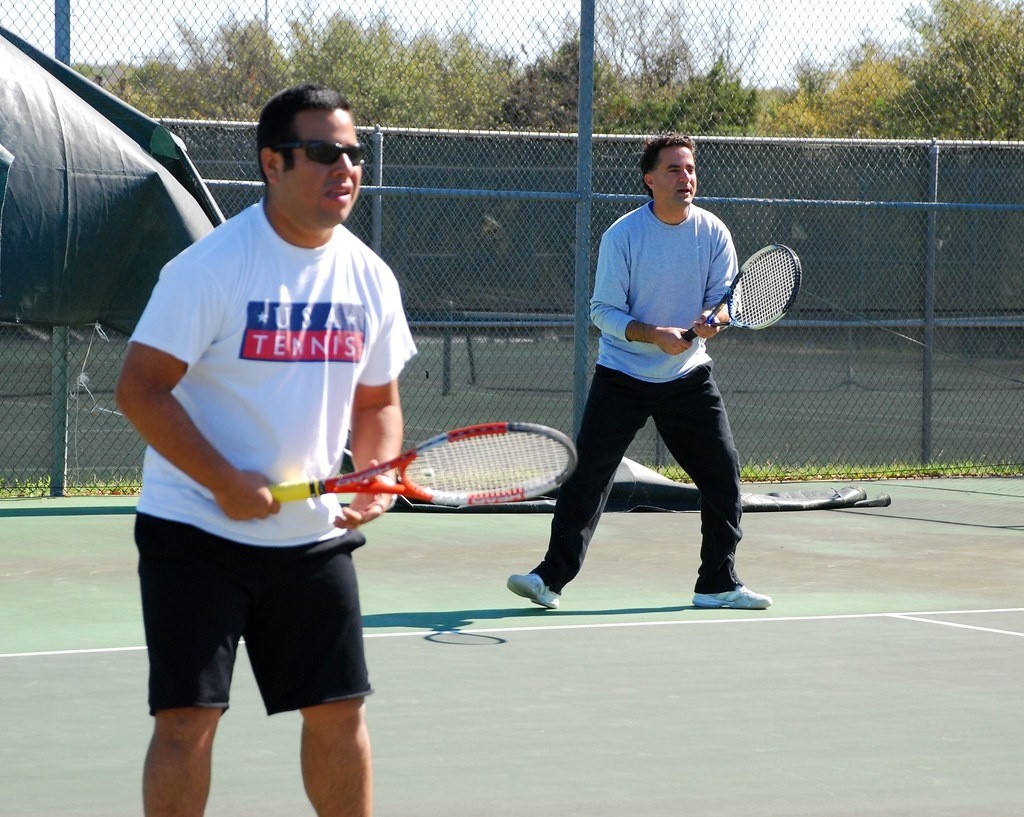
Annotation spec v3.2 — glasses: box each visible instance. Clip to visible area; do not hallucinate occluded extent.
[271,138,367,166]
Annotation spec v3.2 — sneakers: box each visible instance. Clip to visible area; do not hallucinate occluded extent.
[691,584,772,609]
[507,572,564,610]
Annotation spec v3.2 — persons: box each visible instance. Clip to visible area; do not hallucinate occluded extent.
[508,131,772,612]
[116,83,418,817]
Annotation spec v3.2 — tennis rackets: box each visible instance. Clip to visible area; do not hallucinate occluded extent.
[682,243,803,342]
[269,421,579,508]
